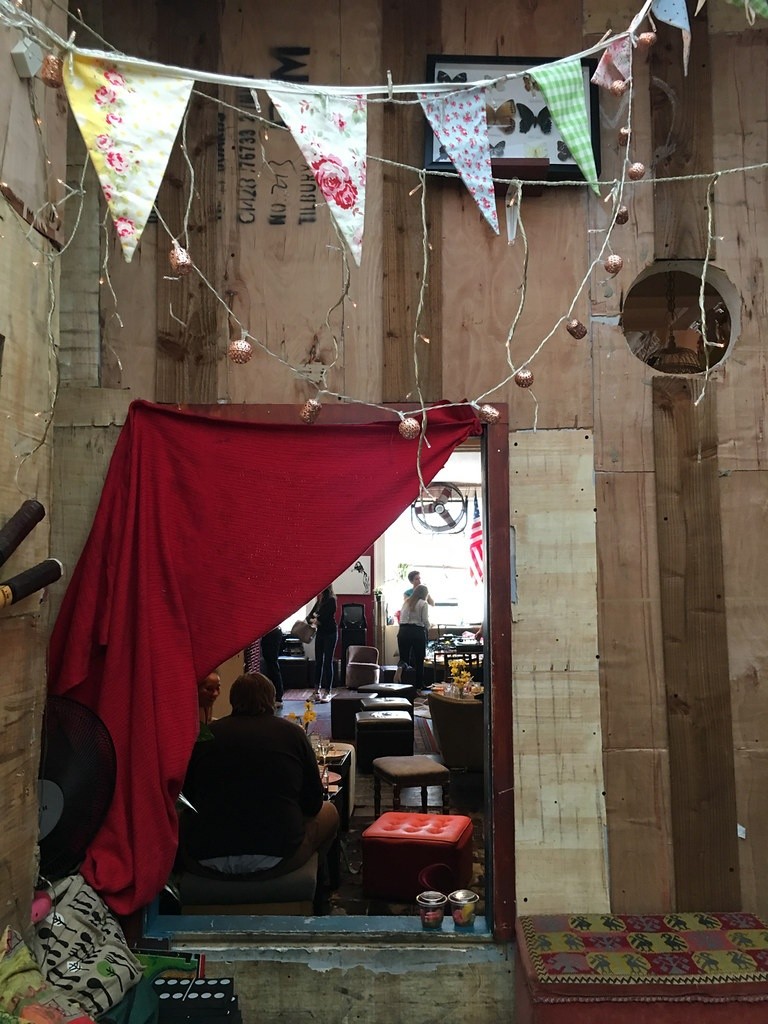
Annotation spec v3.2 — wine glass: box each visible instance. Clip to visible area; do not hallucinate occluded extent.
[321,739,331,765]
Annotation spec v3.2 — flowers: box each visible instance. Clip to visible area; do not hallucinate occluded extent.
[285,699,317,736]
[450,658,473,691]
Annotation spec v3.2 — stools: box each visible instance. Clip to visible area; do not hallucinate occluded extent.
[373,755,450,818]
[331,693,380,741]
[361,812,474,903]
[159,851,318,915]
[360,697,415,743]
[357,683,415,719]
[512,913,768,1024]
[379,664,416,684]
[354,710,413,775]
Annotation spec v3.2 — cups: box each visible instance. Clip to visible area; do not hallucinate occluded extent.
[415,891,449,929]
[311,737,321,765]
[448,888,480,928]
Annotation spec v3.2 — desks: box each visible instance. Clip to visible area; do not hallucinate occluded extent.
[434,652,484,683]
[280,701,331,738]
[428,692,484,771]
[317,750,351,828]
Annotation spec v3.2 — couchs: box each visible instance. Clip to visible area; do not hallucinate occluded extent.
[345,646,380,690]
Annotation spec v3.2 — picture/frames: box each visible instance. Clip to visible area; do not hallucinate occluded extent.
[422,55,601,200]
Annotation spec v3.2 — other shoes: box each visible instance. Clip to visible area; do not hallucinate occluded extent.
[306,691,321,703]
[394,667,402,683]
[274,702,283,709]
[417,689,428,699]
[323,693,331,698]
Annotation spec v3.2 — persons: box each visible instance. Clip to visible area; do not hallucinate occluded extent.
[198,670,221,728]
[404,570,435,607]
[183,670,341,915]
[391,584,432,696]
[304,584,338,702]
[474,621,484,642]
[261,627,285,706]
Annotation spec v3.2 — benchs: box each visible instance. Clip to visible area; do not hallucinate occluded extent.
[423,627,484,686]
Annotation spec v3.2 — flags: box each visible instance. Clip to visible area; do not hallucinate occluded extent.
[469,491,484,586]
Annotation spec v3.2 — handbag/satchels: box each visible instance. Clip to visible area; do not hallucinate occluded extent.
[291,618,317,644]
[29,875,149,1024]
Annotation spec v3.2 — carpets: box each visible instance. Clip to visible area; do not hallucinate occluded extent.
[281,702,444,759]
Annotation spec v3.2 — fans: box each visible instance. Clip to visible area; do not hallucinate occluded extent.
[411,483,465,532]
